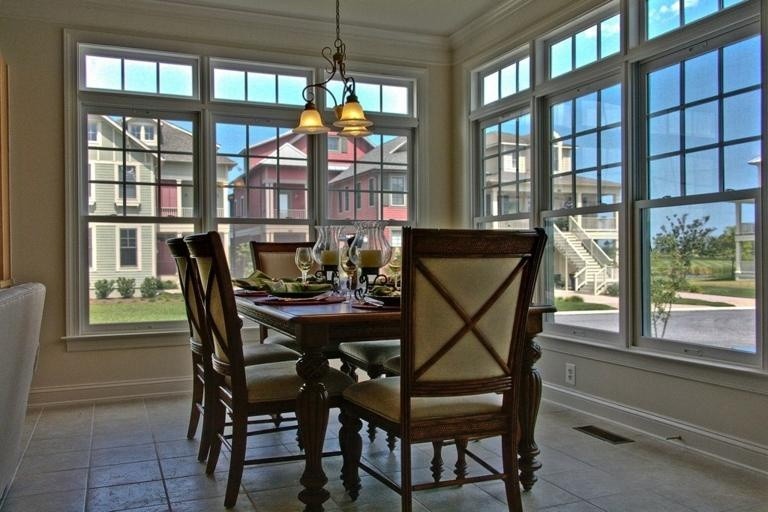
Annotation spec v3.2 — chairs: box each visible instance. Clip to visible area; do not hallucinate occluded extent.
[251,239,331,427]
[384,357,469,487]
[186,230,355,507]
[165,238,301,464]
[339,225,547,512]
[340,340,400,443]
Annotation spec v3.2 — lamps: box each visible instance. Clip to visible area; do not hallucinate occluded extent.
[292,0,373,135]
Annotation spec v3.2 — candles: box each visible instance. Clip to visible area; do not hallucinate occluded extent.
[359,250,381,267]
[321,250,338,265]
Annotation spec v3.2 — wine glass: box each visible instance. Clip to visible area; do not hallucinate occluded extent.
[340,246,358,304]
[388,246,402,290]
[294,247,314,283]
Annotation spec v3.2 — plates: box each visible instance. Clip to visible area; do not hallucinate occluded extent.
[271,291,325,298]
[235,283,269,294]
[368,295,401,306]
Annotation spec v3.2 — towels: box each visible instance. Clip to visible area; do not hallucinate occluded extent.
[232,269,294,290]
[260,278,332,294]
[368,274,401,284]
[370,285,394,295]
